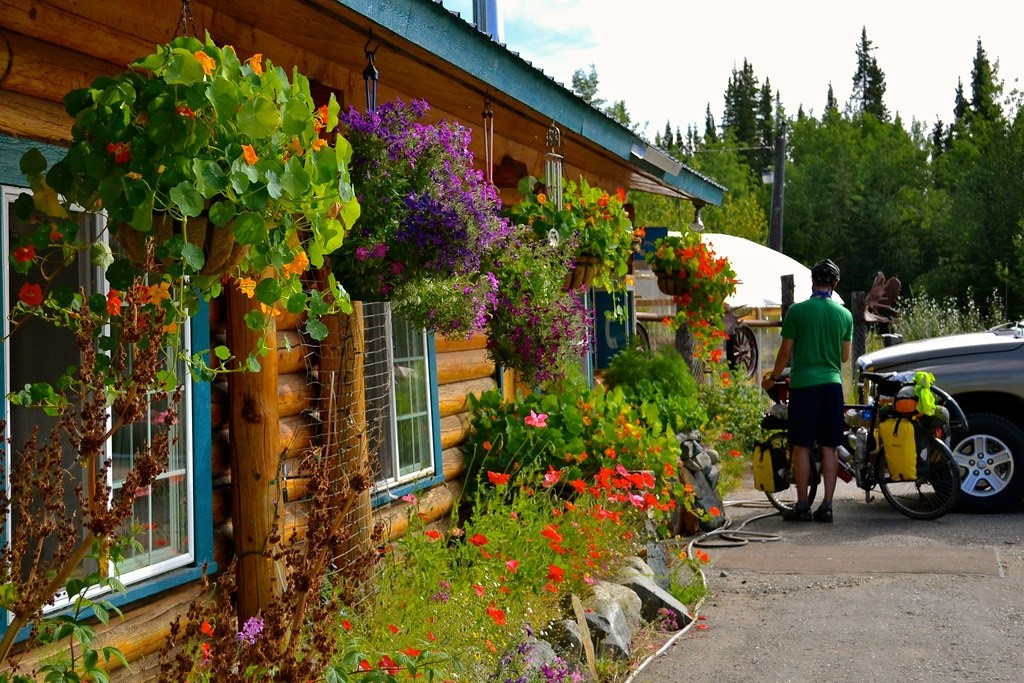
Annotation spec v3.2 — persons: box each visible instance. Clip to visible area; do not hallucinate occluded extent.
[761,258,854,523]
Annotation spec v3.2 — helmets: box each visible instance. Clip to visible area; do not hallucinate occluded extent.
[811,258,841,289]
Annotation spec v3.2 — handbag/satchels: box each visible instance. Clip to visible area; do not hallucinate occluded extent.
[752,439,790,493]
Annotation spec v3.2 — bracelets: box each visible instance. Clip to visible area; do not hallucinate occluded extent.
[770,375,776,381]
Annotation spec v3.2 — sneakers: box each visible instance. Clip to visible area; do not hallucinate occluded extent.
[811,507,834,524]
[782,505,813,522]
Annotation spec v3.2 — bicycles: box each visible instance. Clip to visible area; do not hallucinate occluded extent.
[753,364,968,521]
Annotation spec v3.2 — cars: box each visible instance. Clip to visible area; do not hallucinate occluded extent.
[854,314,1024,514]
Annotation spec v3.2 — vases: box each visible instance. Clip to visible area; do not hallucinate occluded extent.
[332,250,380,303]
[104,205,254,282]
[563,255,603,291]
[655,269,689,295]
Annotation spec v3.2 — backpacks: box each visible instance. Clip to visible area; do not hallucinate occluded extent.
[894,386,919,415]
[879,416,922,482]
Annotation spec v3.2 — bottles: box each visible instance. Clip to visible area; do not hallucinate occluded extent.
[854,426,868,463]
[835,445,852,462]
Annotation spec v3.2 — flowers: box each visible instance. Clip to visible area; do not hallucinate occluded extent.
[16,32,734,388]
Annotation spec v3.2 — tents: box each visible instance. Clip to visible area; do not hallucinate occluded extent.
[701,234,844,309]
